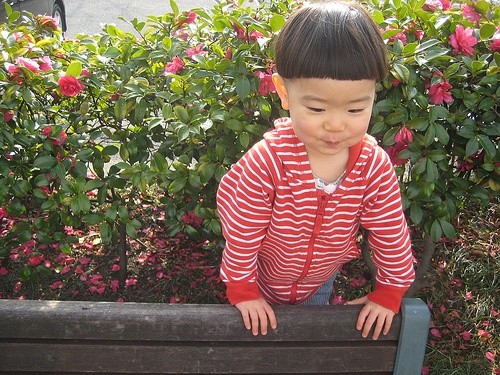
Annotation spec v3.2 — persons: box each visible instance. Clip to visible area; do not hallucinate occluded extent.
[216,0,416,341]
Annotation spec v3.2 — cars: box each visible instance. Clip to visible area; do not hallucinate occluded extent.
[0,0,67,43]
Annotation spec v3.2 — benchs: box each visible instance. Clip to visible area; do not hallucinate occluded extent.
[0,284,431,375]
